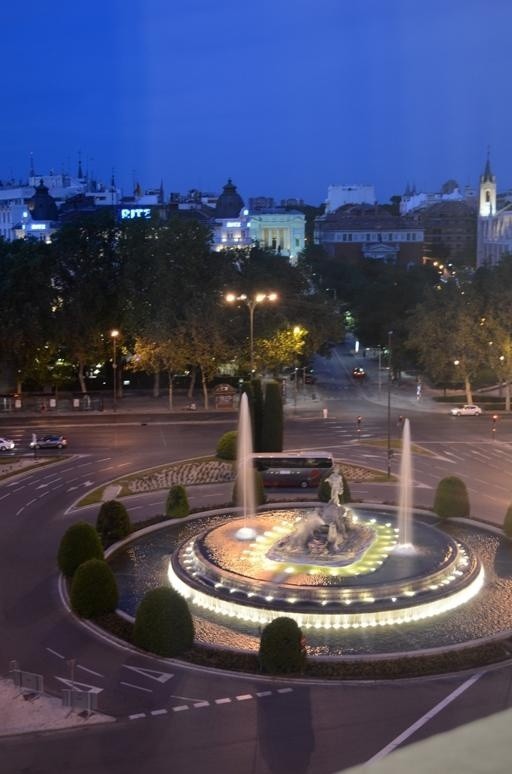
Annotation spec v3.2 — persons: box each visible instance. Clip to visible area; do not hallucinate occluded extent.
[323,408,328,419]
[357,416,362,431]
[398,415,403,431]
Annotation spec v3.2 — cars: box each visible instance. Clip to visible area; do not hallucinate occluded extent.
[0,437,15,452]
[289,367,319,385]
[352,367,367,379]
[450,404,483,417]
[29,433,68,450]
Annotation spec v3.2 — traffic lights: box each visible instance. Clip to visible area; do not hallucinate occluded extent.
[492,415,498,419]
[357,415,363,422]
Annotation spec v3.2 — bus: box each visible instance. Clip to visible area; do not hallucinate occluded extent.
[251,451,336,489]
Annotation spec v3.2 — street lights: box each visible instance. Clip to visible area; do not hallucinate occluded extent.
[221,287,280,382]
[110,328,121,408]
